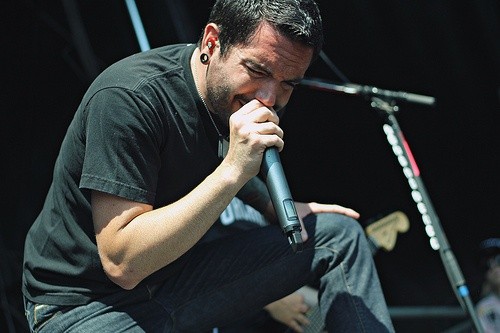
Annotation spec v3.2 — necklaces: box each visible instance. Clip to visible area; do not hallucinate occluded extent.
[193,47,230,140]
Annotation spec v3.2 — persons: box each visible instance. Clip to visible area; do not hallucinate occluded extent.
[23,0,396,333]
[218,196,322,333]
[474,239,500,333]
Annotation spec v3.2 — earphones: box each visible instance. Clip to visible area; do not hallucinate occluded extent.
[206,37,217,55]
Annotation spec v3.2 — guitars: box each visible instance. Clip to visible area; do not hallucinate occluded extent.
[219,210,410,333]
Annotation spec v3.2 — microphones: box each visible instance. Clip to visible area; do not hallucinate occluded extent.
[258,120,305,250]
